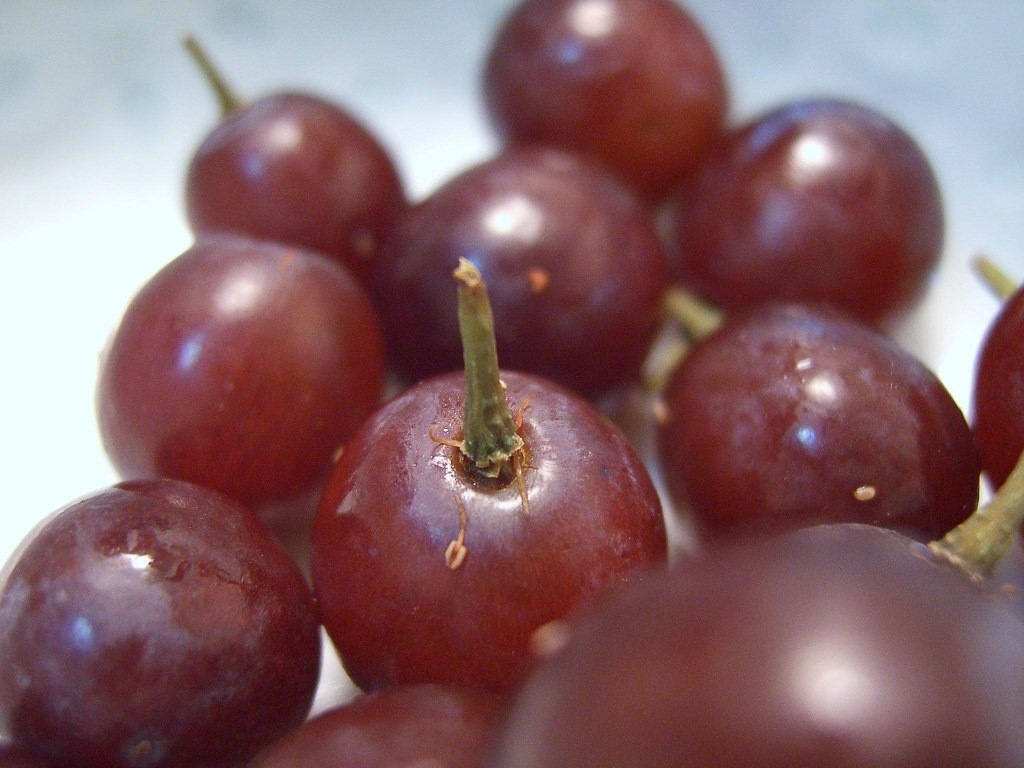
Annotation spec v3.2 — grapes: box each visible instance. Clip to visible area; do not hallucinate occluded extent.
[0,0,1024,767]
